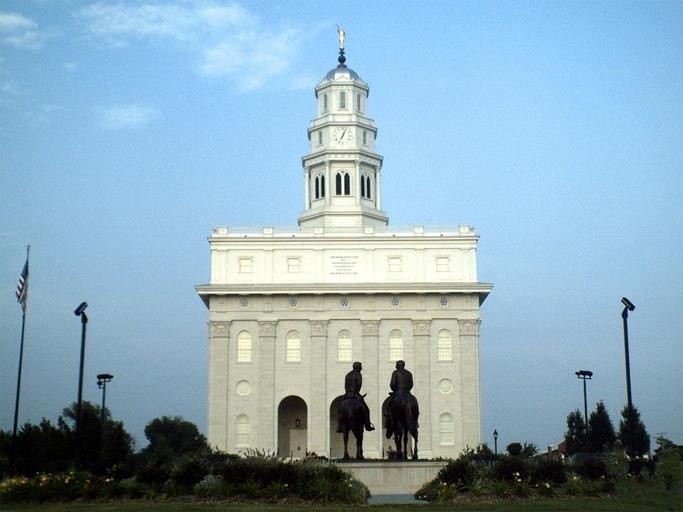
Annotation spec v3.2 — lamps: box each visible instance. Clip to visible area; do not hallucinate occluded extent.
[295,417,300,428]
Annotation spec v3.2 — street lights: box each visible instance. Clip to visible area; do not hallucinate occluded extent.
[492,429,498,455]
[74,301,90,432]
[95,374,115,417]
[575,369,593,430]
[620,295,635,404]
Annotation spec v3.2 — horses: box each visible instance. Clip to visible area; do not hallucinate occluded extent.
[336,391,369,460]
[383,391,420,458]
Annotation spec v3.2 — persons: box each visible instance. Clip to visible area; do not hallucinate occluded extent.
[383,360,419,439]
[336,361,375,433]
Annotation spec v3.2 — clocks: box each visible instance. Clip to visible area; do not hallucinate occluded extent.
[332,127,352,145]
